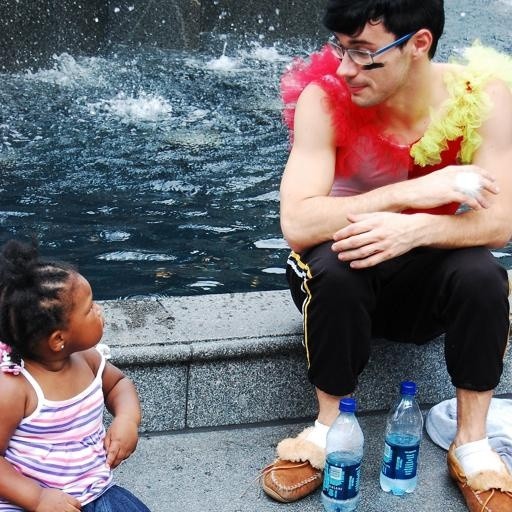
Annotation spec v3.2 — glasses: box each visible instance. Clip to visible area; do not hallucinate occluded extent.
[327,32,415,66]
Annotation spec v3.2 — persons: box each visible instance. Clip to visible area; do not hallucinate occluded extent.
[0,234,152,512]
[262,0,512,512]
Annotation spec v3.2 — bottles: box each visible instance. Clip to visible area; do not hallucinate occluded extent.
[322,399,364,512]
[378,383,422,498]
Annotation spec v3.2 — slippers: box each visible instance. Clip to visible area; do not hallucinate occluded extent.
[447,440,512,512]
[262,426,327,503]
